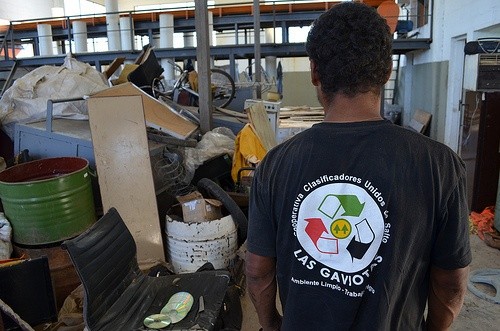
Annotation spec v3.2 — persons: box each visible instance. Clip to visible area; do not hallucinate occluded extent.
[244,2,473,331]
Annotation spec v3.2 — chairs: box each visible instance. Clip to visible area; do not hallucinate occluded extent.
[61,207,231,331]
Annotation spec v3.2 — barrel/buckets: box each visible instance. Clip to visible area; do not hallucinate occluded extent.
[165,200,238,275]
[0,156,96,310]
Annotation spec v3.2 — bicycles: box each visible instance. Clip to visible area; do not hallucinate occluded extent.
[137,59,236,113]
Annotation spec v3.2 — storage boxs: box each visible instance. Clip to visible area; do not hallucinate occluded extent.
[176,192,223,222]
[244,99,282,136]
